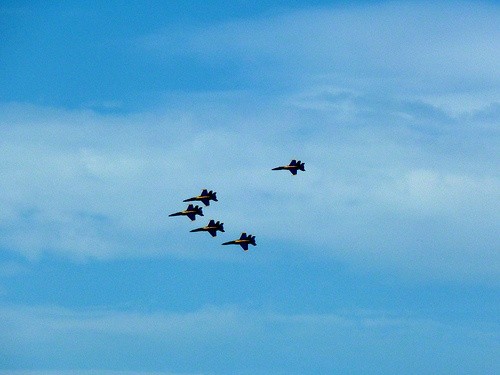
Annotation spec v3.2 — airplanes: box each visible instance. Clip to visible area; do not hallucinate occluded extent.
[221,232,257,252]
[182,189,219,207]
[270,160,305,175]
[189,219,225,238]
[168,203,204,221]
